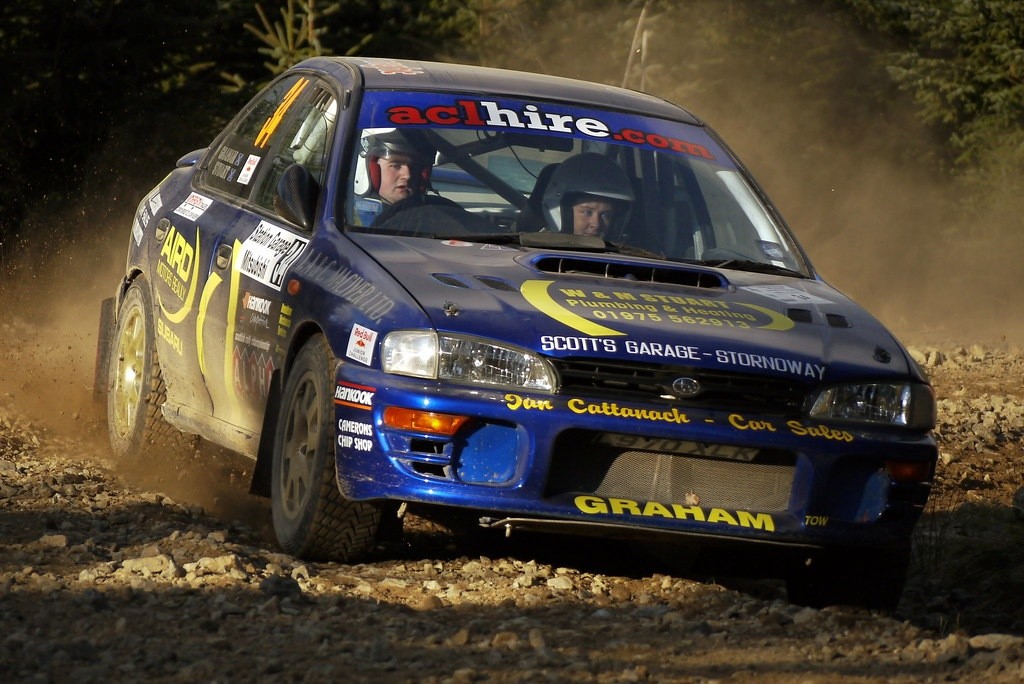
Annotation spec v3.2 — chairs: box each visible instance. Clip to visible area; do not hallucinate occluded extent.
[515,160,559,234]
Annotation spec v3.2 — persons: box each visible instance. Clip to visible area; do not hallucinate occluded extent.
[540,153,636,252]
[353,127,437,230]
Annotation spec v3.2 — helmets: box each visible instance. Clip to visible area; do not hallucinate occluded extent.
[355,128,436,198]
[543,153,635,246]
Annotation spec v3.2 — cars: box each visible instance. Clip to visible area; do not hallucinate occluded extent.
[108,58,942,612]
